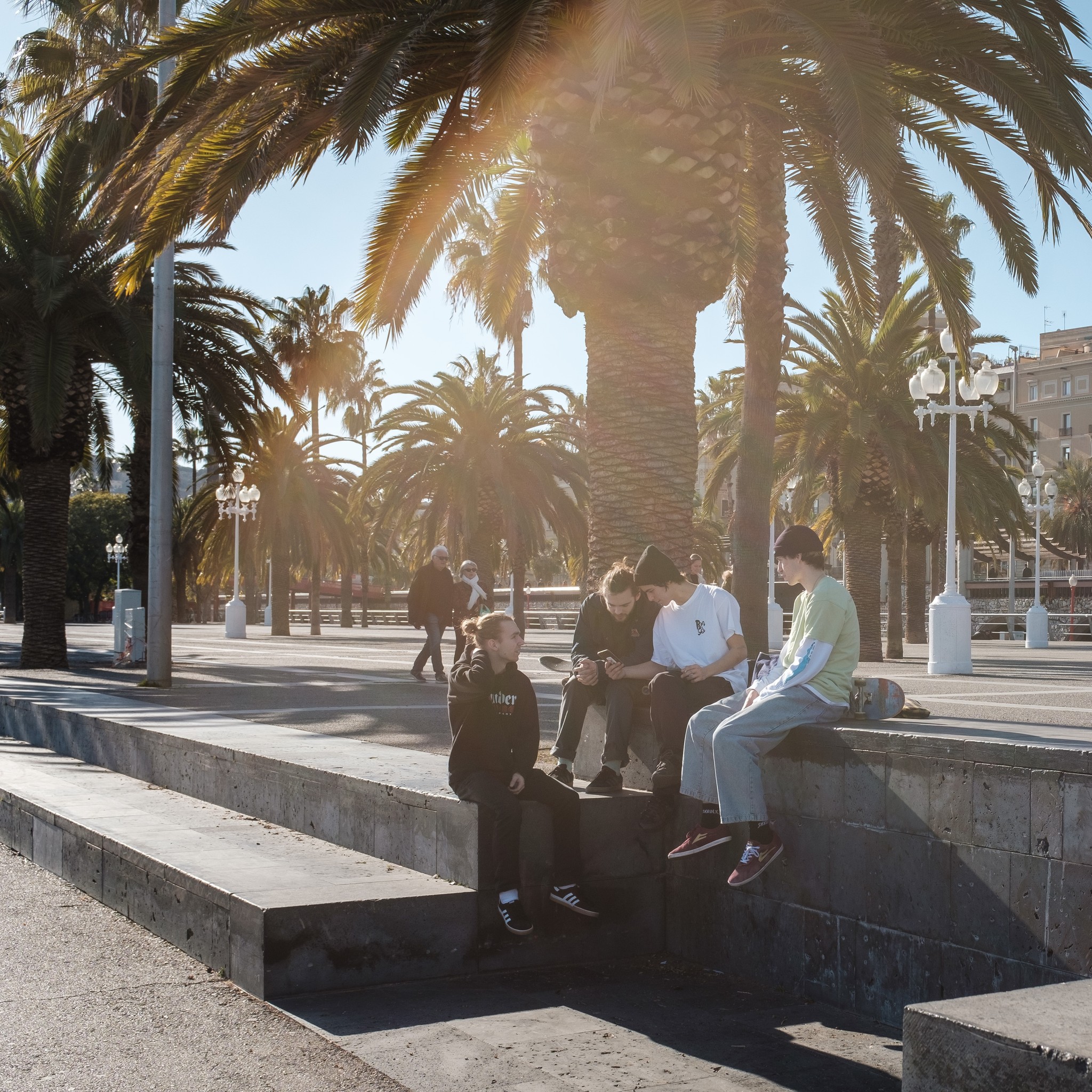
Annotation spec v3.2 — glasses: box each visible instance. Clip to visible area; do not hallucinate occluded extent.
[464,568,477,572]
[434,555,449,561]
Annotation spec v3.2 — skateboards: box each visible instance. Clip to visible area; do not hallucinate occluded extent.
[538,656,574,687]
[849,677,905,722]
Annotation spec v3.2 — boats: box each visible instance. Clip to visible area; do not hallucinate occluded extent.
[295,572,388,601]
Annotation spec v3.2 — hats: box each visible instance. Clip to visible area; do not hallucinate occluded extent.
[633,545,680,586]
[773,525,824,556]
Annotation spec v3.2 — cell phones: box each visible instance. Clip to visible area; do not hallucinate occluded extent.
[598,649,626,668]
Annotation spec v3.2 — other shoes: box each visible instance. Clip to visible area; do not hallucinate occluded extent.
[638,786,680,830]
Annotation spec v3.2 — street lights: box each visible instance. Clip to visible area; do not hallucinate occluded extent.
[768,473,800,650]
[1015,457,1059,650]
[906,317,1002,674]
[215,464,260,636]
[1068,573,1078,643]
[105,533,129,590]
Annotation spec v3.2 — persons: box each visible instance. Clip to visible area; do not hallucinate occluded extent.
[605,545,749,794]
[685,553,706,585]
[720,563,733,592]
[452,560,490,666]
[446,612,602,934]
[549,560,667,792]
[404,545,453,683]
[971,628,992,640]
[494,575,569,588]
[988,564,998,581]
[1022,563,1032,578]
[668,525,860,887]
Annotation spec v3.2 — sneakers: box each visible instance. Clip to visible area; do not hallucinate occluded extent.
[435,674,449,683]
[497,897,534,935]
[667,821,733,860]
[727,829,784,888]
[585,765,623,793]
[410,669,426,682]
[547,764,574,789]
[549,884,599,918]
